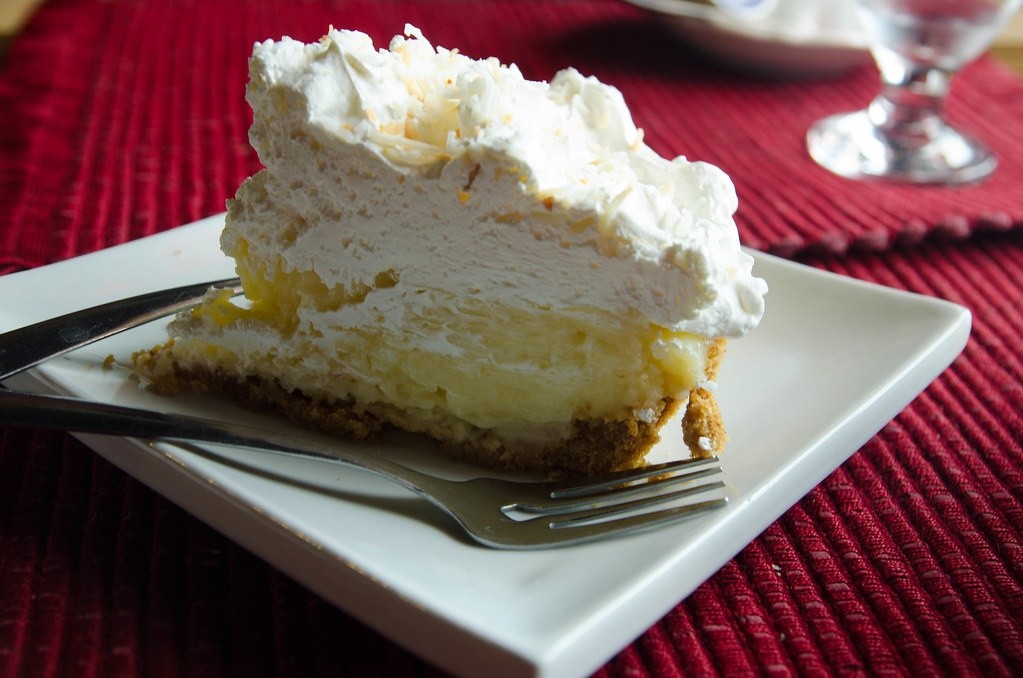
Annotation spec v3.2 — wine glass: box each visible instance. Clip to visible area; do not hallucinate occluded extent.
[807,0,1020,182]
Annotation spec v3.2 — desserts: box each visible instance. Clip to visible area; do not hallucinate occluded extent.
[102,24,770,489]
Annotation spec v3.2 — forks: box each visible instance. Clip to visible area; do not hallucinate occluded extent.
[1,386,733,550]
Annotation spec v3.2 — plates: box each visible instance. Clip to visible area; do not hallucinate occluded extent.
[0,181,976,678]
[631,0,875,79]
